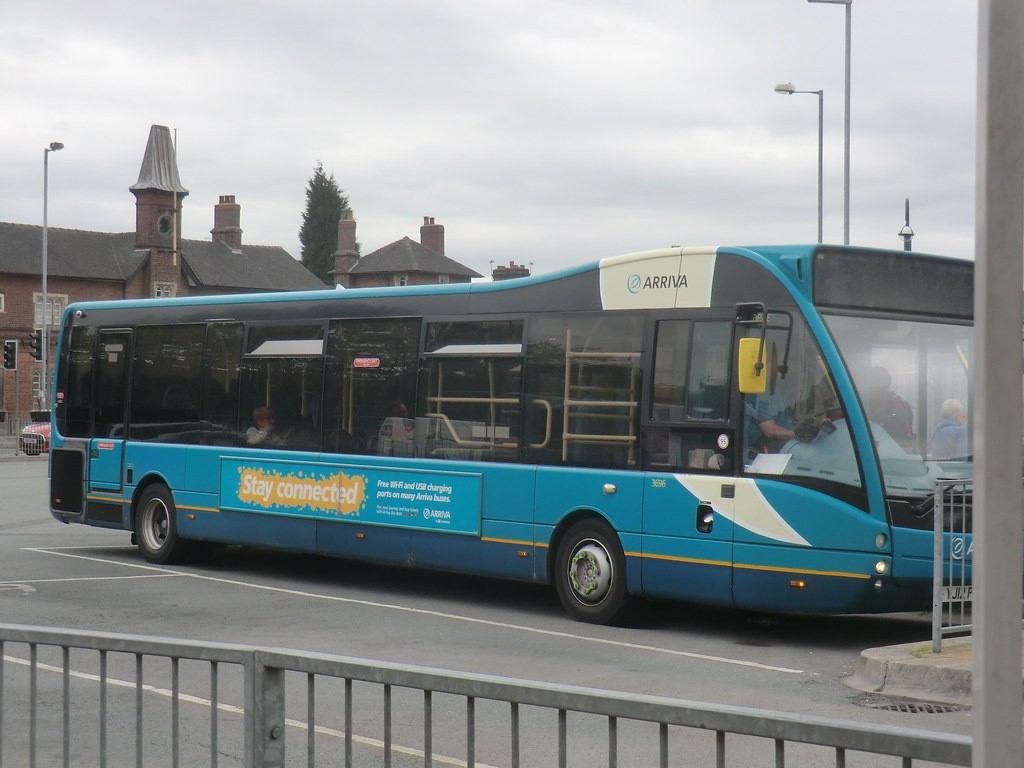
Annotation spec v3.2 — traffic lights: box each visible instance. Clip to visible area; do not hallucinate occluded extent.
[4,341,18,371]
[29,328,43,363]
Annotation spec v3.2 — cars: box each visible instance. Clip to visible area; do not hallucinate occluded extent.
[19,420,51,456]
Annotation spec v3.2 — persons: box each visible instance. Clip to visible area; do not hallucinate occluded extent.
[380,402,413,441]
[244,406,284,448]
[744,343,916,454]
[929,398,967,460]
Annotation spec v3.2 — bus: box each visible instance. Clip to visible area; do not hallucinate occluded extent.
[48,240,974,624]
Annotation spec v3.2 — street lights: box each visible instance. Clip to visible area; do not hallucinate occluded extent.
[42,142,64,412]
[807,0,852,246]
[774,82,823,245]
[897,197,915,250]
